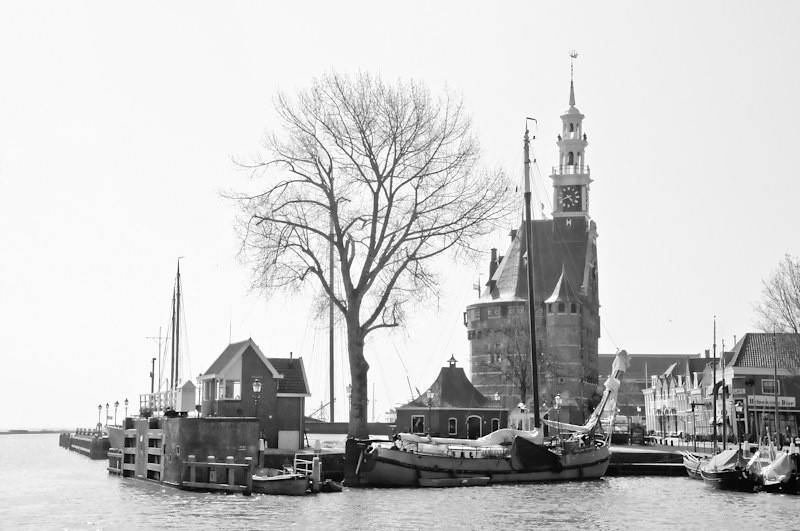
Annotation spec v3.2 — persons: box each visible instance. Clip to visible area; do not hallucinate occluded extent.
[772,429,783,451]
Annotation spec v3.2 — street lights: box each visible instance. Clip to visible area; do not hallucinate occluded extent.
[123,398,129,417]
[113,401,120,426]
[151,357,157,393]
[98,404,103,436]
[105,403,109,426]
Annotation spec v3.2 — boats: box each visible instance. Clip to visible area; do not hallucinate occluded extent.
[253,464,312,497]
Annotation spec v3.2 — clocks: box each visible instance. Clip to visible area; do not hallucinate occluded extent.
[558,187,580,208]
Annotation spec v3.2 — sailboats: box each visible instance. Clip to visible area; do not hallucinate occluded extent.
[343,116,633,489]
[679,316,800,495]
